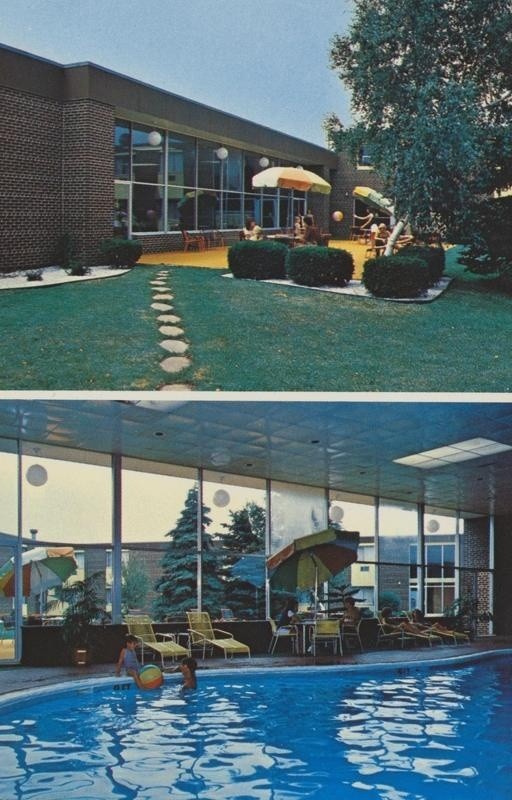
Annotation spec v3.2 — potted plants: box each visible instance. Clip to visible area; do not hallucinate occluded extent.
[58,568,111,665]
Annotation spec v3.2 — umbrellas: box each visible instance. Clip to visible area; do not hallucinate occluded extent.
[252,165,331,234]
[175,190,219,214]
[351,185,395,220]
[1,545,78,618]
[266,528,359,619]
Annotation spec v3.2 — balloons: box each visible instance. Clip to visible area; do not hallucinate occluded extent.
[296,165,303,168]
[119,132,133,145]
[426,519,439,532]
[259,158,269,168]
[28,464,49,487]
[213,489,229,507]
[329,507,343,521]
[149,132,161,145]
[217,148,228,159]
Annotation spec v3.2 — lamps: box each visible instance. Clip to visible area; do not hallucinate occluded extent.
[328,489,345,523]
[25,443,49,488]
[425,504,441,534]
[213,473,230,509]
[294,163,304,172]
[148,127,163,147]
[258,154,270,168]
[215,144,228,160]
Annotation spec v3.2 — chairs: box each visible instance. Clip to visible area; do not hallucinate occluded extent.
[180,218,417,264]
[266,610,470,657]
[127,614,188,669]
[187,610,251,661]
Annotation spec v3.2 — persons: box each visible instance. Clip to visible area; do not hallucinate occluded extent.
[409,609,455,634]
[242,208,391,257]
[277,598,303,654]
[381,607,429,638]
[135,215,144,231]
[116,635,144,689]
[180,658,197,690]
[341,597,360,624]
[269,208,272,223]
[148,207,158,230]
